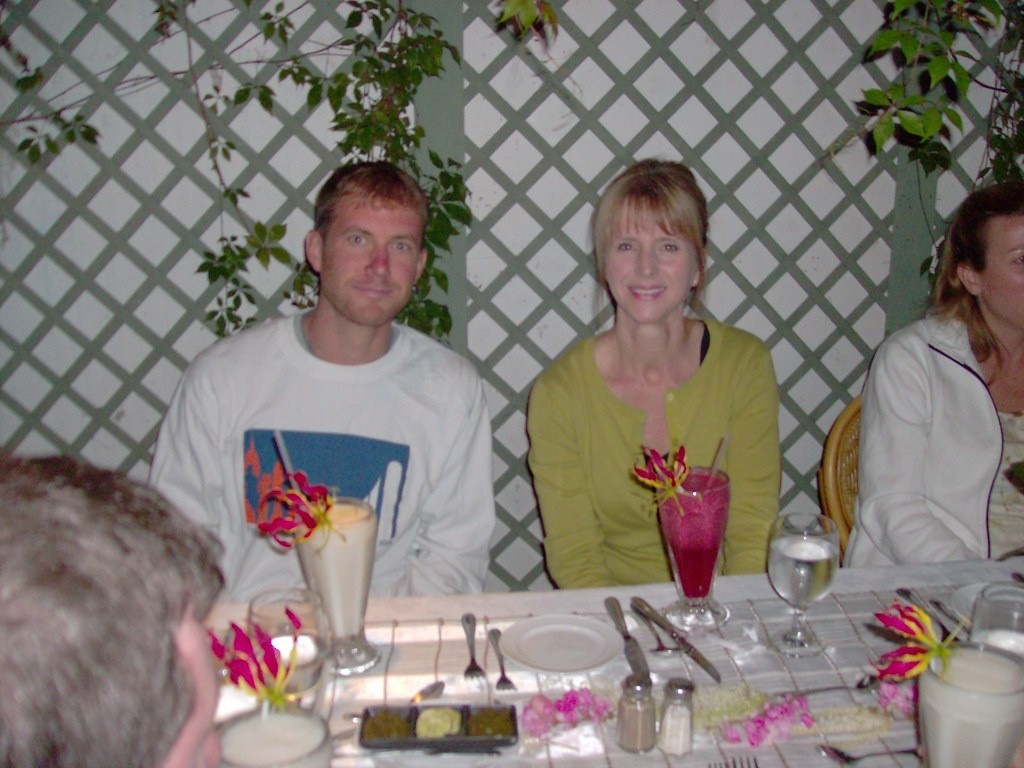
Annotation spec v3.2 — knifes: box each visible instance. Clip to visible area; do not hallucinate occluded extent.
[631,596,722,683]
[604,595,650,682]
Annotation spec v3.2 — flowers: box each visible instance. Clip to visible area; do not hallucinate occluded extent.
[870,597,966,685]
[252,471,349,556]
[208,602,307,712]
[634,442,703,516]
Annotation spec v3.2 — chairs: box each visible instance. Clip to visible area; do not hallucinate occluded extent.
[821,396,860,560]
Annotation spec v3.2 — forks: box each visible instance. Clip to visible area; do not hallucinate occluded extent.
[461,612,489,688]
[487,630,517,696]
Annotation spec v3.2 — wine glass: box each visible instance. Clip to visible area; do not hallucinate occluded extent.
[766,512,839,658]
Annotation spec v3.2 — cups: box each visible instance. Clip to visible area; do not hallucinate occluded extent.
[917,640,1024,768]
[247,587,330,696]
[967,581,1024,659]
[216,707,334,768]
[656,465,731,633]
[291,497,381,676]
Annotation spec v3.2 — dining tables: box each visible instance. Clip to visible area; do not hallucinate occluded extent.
[209,558,1024,766]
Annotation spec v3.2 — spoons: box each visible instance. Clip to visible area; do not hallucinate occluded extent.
[815,743,917,763]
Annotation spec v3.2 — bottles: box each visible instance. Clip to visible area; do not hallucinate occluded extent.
[615,674,657,754]
[657,678,695,757]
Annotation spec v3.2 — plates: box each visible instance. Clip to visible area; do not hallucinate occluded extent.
[950,581,991,625]
[498,613,625,678]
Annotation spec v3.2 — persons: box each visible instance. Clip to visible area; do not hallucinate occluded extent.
[0,448,229,768]
[839,171,1024,570]
[143,159,496,606]
[527,159,787,589]
